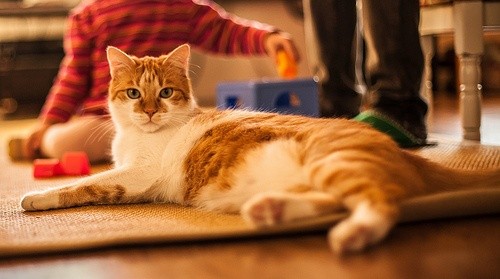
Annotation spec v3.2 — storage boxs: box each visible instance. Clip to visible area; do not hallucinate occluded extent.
[215,79,320,118]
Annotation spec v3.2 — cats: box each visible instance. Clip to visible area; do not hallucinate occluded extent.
[19,42,500,253]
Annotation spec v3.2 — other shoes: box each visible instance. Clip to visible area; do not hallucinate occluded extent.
[351,110,427,148]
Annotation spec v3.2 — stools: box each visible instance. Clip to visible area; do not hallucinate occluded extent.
[417,0,500,142]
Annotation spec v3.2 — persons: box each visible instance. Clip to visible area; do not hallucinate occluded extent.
[302,0,429,148]
[9,0,310,162]
